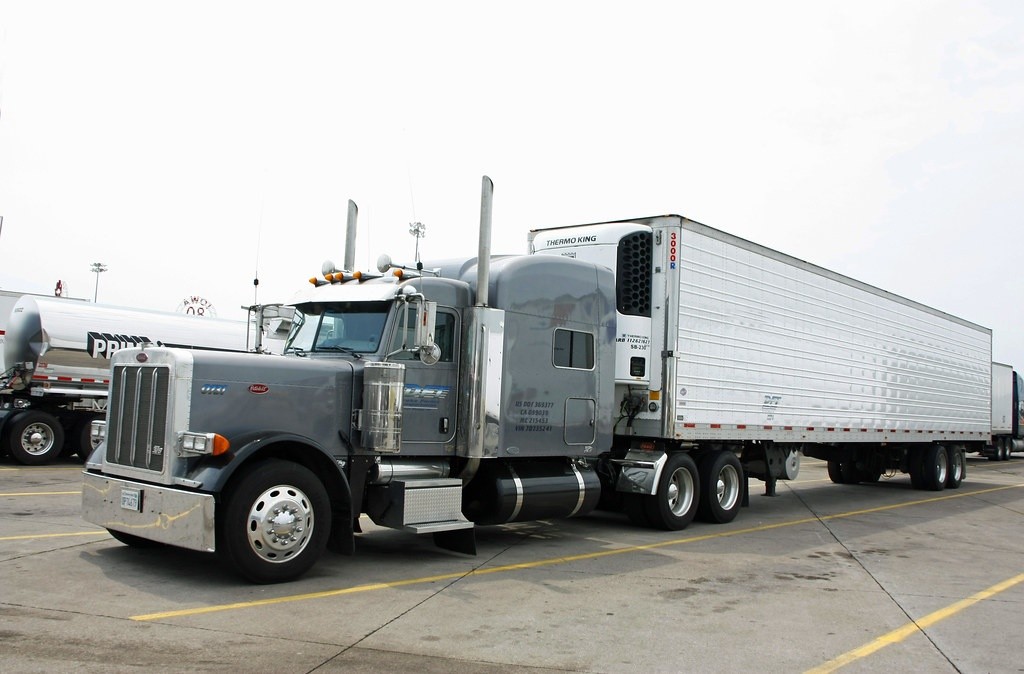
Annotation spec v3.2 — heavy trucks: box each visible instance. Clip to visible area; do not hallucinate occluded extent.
[0,290,338,466]
[965,362,1024,461]
[82,176,993,585]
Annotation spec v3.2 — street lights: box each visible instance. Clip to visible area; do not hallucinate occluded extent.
[90,262,108,303]
[409,220,427,263]
